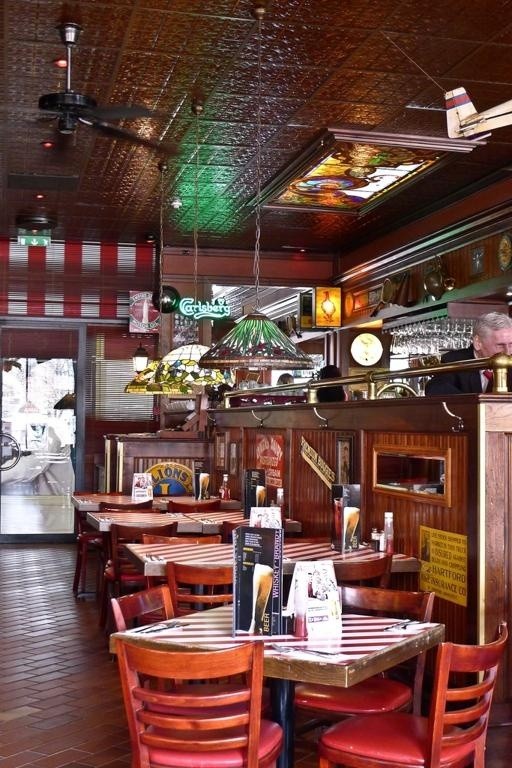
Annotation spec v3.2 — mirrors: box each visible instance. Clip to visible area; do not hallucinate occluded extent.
[202,277,341,390]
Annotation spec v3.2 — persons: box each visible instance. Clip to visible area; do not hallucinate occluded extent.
[305,568,334,599]
[342,446,349,484]
[276,374,294,386]
[254,513,279,528]
[425,312,511,396]
[135,477,151,489]
[317,365,347,404]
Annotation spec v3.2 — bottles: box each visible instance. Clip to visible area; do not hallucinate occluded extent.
[276,488,286,529]
[308,572,313,598]
[293,570,308,637]
[378,529,386,552]
[370,527,379,540]
[221,473,231,502]
[383,511,395,556]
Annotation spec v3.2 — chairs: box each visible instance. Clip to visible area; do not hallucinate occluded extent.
[73,491,511,768]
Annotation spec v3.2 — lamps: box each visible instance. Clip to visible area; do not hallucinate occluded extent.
[125,7,315,395]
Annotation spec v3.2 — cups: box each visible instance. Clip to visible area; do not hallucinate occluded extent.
[247,563,274,635]
[255,485,266,507]
[196,472,209,501]
[343,506,360,552]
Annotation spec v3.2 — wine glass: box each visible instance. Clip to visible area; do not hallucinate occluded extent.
[388,317,476,355]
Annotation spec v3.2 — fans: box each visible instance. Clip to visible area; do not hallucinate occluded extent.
[1,22,186,156]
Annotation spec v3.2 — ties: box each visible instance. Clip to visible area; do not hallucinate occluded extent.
[483,370,494,393]
